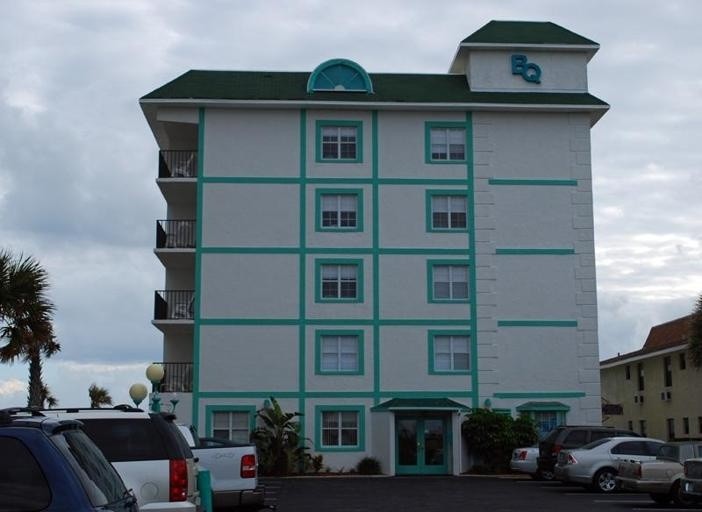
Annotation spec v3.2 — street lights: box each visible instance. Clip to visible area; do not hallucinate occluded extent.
[129,363,178,412]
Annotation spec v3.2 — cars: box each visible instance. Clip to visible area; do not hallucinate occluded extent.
[1,404,259,512]
[510,425,701,508]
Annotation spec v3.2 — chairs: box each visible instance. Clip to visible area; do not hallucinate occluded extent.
[163,150,195,393]
